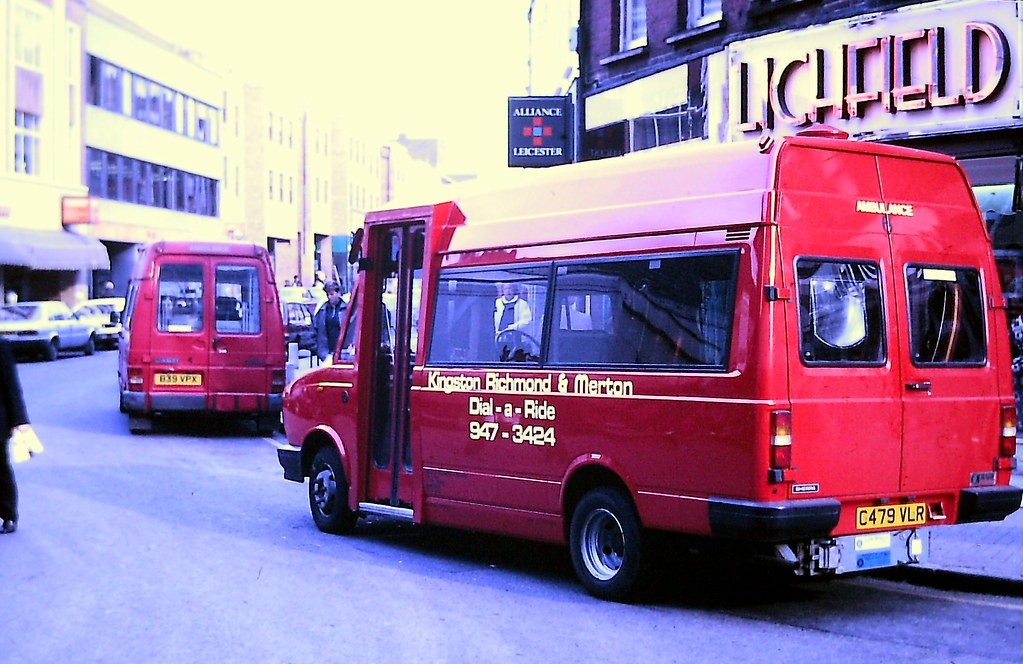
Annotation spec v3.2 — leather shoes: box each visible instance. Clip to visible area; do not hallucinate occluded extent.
[0,514,19,534]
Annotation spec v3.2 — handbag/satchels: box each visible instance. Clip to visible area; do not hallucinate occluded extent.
[4,427,43,463]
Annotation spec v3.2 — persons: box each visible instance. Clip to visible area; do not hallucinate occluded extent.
[311,274,325,288]
[292,275,304,287]
[490,282,532,342]
[559,295,594,331]
[311,280,353,361]
[0,330,45,537]
[284,280,291,286]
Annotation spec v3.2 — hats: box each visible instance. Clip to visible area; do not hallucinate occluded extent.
[323,281,339,294]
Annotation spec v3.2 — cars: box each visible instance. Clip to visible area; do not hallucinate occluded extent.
[1,300,98,361]
[275,273,420,344]
[72,298,126,347]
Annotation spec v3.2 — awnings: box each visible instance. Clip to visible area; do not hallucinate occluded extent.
[0,228,111,271]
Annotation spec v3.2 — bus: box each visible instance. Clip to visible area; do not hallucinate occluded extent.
[270,124,1023,599]
[119,241,284,438]
